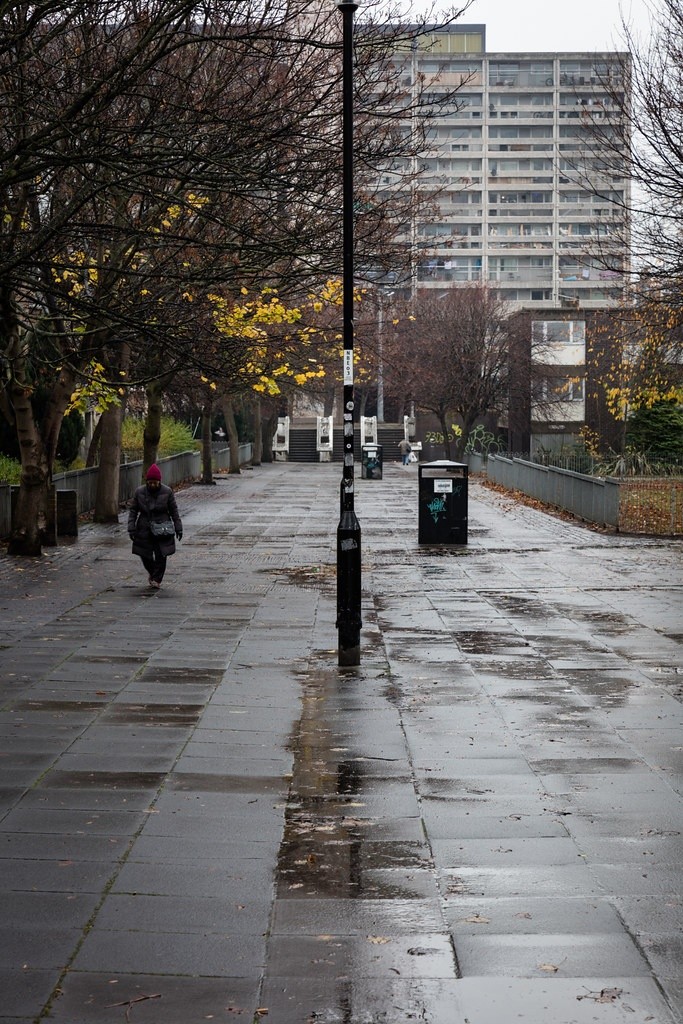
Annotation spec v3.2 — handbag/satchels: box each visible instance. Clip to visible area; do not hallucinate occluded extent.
[150,521,175,536]
[406,444,411,452]
[409,452,417,463]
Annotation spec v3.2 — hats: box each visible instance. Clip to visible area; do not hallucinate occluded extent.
[146,464,161,480]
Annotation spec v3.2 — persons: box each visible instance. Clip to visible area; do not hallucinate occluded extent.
[127,464,183,589]
[398,437,411,466]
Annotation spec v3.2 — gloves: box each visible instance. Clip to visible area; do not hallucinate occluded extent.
[129,531,136,541]
[176,531,183,541]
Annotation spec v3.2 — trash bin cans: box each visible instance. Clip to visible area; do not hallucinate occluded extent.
[419,460,468,545]
[362,445,383,480]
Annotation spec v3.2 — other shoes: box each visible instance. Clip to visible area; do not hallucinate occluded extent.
[149,575,160,588]
[403,462,408,465]
